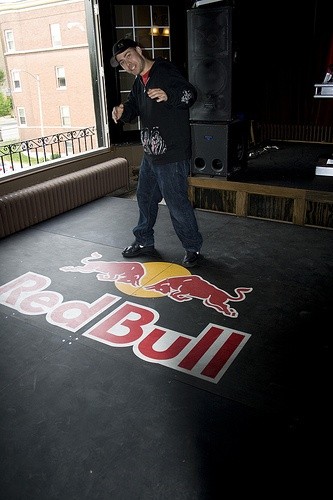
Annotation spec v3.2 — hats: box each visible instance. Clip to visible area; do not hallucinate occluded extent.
[113,38,136,56]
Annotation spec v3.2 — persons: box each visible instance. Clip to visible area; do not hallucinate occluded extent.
[110,37,204,266]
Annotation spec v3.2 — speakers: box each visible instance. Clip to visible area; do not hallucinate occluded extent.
[186,6,239,122]
[190,123,245,182]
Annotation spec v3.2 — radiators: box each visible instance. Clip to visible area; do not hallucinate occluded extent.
[0,156,129,240]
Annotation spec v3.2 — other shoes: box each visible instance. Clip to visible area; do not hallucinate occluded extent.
[182,250,200,268]
[121,239,155,258]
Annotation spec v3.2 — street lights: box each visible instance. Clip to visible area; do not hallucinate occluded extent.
[15,70,44,137]
